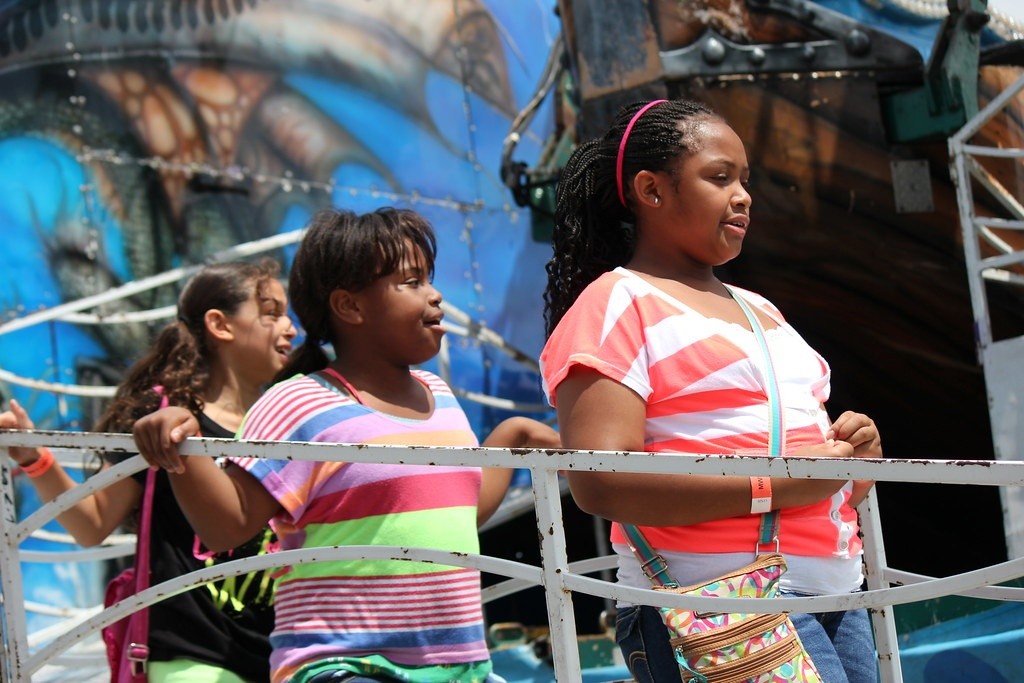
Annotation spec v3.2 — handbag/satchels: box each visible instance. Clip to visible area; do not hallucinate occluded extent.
[653,553,825,683]
[102,569,150,683]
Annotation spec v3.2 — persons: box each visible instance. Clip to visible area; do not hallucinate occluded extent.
[0,262,299,682]
[538,98,884,683]
[133,206,560,682]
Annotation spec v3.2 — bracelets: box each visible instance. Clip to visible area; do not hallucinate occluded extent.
[19,446,55,478]
[749,477,771,514]
